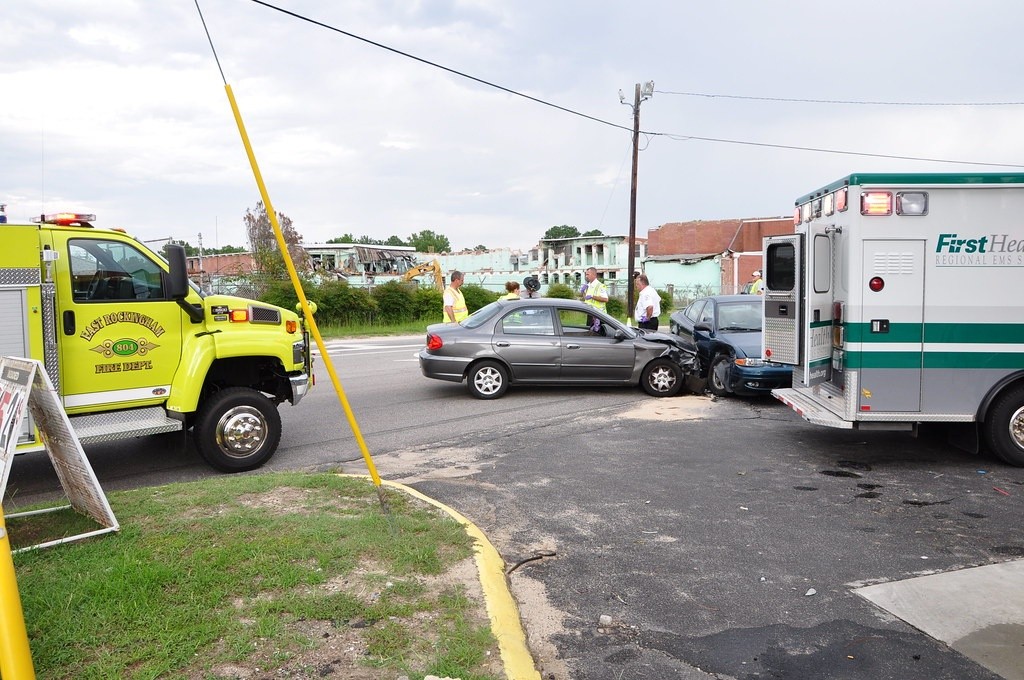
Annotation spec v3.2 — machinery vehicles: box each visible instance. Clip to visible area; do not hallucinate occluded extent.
[400,258,444,294]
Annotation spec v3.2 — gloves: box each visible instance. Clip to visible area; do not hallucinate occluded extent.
[580,284,588,293]
[585,295,592,300]
[640,315,650,323]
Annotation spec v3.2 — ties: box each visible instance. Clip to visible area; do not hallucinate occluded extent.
[529,293,532,298]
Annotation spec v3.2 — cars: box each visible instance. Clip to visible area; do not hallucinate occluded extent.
[419,298,702,401]
[669,294,793,397]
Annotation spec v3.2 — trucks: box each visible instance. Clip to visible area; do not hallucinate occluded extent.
[762,172,1024,467]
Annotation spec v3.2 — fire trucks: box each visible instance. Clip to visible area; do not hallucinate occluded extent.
[0,204,318,473]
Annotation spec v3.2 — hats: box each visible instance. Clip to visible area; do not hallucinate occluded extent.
[751,271,761,276]
[523,277,541,291]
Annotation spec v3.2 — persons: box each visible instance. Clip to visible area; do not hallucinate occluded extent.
[521,277,542,299]
[441,271,470,324]
[757,287,763,296]
[750,271,764,295]
[634,274,662,331]
[581,267,609,327]
[496,281,520,328]
